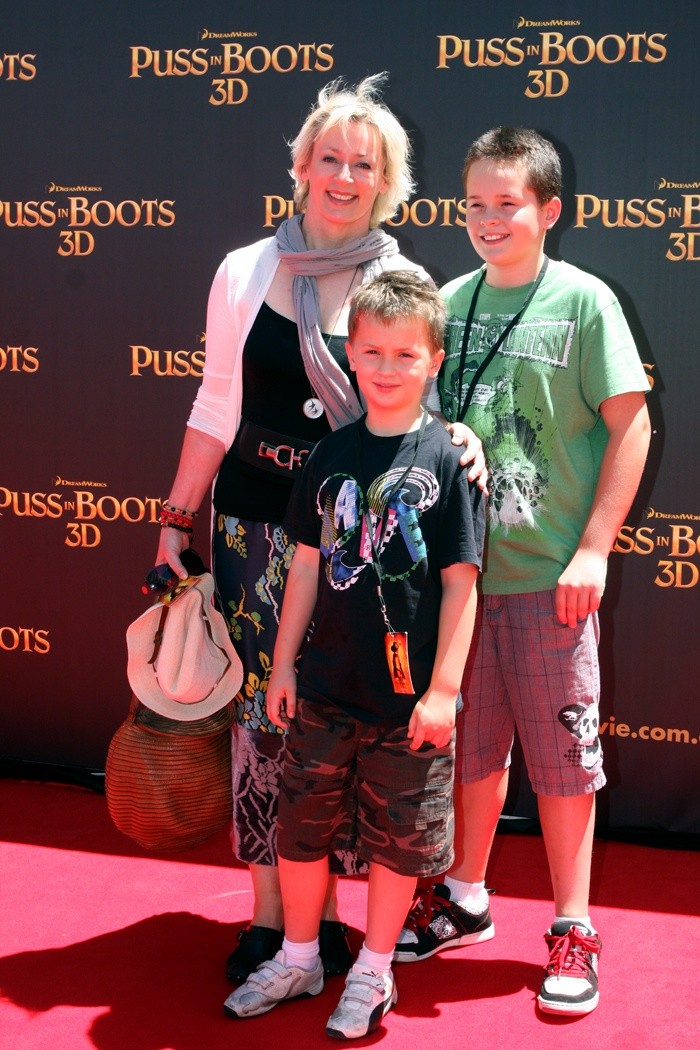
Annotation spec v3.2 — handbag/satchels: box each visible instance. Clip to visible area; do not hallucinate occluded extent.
[105,693,237,852]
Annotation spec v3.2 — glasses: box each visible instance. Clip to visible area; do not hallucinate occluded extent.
[144,548,206,595]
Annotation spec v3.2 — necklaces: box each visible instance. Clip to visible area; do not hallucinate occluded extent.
[303,265,359,419]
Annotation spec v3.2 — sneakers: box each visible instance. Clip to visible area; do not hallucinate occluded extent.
[325,964,397,1040]
[537,920,602,1015]
[223,950,324,1019]
[393,882,495,963]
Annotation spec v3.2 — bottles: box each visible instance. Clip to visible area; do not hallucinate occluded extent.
[139,548,205,595]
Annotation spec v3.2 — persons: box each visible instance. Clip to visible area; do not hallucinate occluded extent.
[153,91,490,988]
[219,269,488,1040]
[384,125,652,1018]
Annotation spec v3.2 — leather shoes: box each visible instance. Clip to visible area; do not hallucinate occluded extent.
[318,920,354,975]
[224,925,282,985]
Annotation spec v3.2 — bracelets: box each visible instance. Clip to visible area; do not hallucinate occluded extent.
[158,499,199,534]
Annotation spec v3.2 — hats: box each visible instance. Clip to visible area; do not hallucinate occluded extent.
[125,573,244,722]
